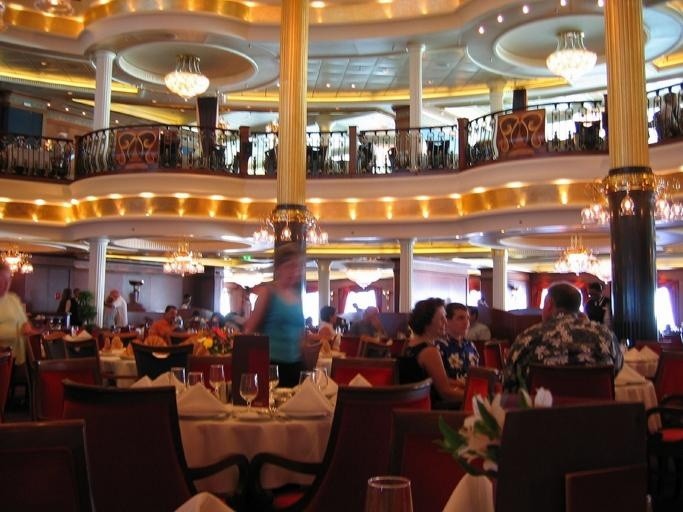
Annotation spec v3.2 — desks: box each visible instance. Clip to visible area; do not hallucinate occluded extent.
[6,144,52,175]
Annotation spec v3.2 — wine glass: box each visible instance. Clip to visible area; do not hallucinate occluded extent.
[168,362,330,421]
[621,338,630,352]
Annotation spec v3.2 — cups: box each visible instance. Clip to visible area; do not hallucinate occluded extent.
[363,474,415,511]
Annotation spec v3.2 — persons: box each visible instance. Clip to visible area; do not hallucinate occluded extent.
[498,285,624,392]
[584,282,612,325]
[396,298,492,411]
[308,306,384,375]
[1,266,252,409]
[355,134,376,174]
[233,242,308,389]
[643,92,682,144]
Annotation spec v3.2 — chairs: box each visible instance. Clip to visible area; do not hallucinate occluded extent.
[0,323,683,512]
[162,133,277,176]
[307,121,604,174]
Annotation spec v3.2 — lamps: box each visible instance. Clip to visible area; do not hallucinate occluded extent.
[164,55,209,101]
[581,173,683,227]
[162,241,206,276]
[253,205,328,249]
[564,235,598,277]
[0,244,33,275]
[545,30,598,86]
